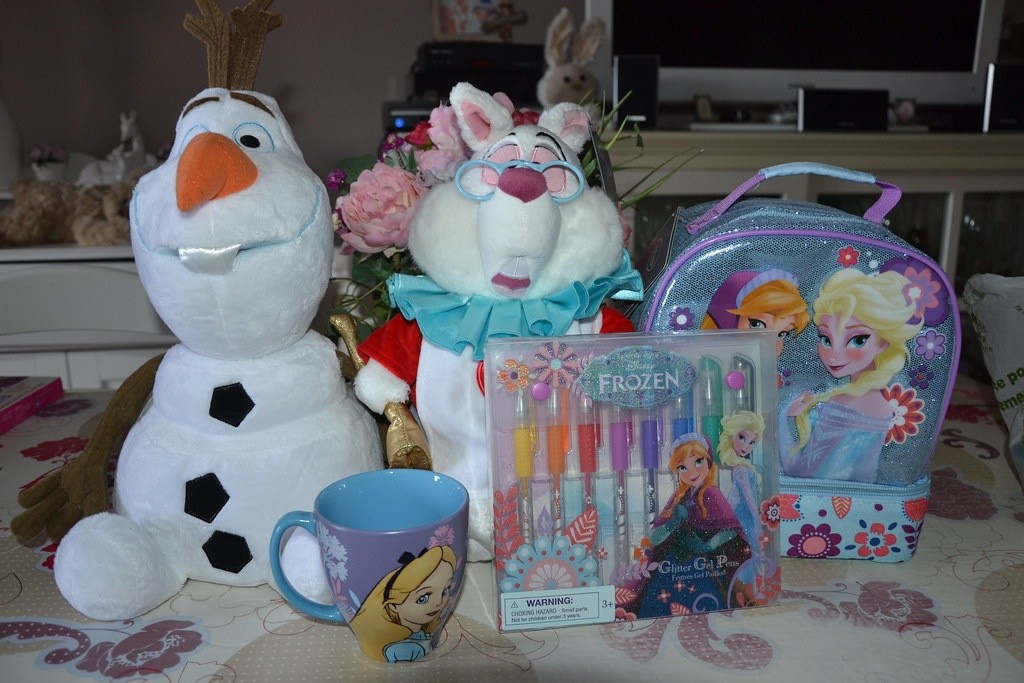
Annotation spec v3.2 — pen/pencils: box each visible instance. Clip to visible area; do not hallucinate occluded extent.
[502,353,769,617]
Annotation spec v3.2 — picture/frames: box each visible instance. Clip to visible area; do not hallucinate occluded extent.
[431,1,507,42]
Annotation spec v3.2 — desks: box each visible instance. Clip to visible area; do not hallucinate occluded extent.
[0,300,1023,683]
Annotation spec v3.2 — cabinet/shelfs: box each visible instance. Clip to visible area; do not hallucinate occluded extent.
[600,129,1023,287]
[0,247,181,392]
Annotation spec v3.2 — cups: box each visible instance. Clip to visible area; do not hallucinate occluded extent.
[270,468,470,663]
